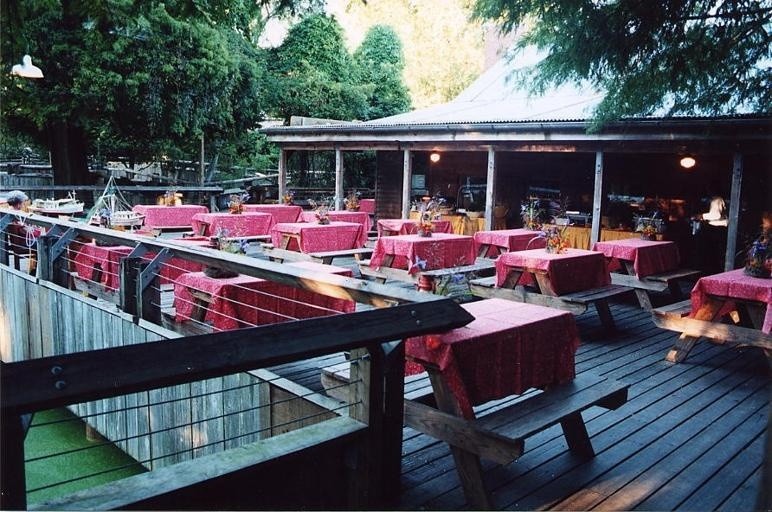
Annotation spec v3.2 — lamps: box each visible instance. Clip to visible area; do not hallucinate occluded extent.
[678,156,696,169]
[428,151,440,162]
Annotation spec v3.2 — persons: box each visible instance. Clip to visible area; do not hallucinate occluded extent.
[694,187,728,260]
[6,196,37,276]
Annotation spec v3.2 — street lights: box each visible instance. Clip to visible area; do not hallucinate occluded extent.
[0,0,47,97]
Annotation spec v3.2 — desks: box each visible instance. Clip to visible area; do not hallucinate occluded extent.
[30,207,83,218]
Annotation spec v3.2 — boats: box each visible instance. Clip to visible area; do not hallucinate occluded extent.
[32,186,86,214]
[85,165,146,231]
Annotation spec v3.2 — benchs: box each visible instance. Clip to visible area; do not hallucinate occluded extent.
[73,192,772,512]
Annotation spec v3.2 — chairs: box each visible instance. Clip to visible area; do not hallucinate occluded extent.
[5,224,37,270]
